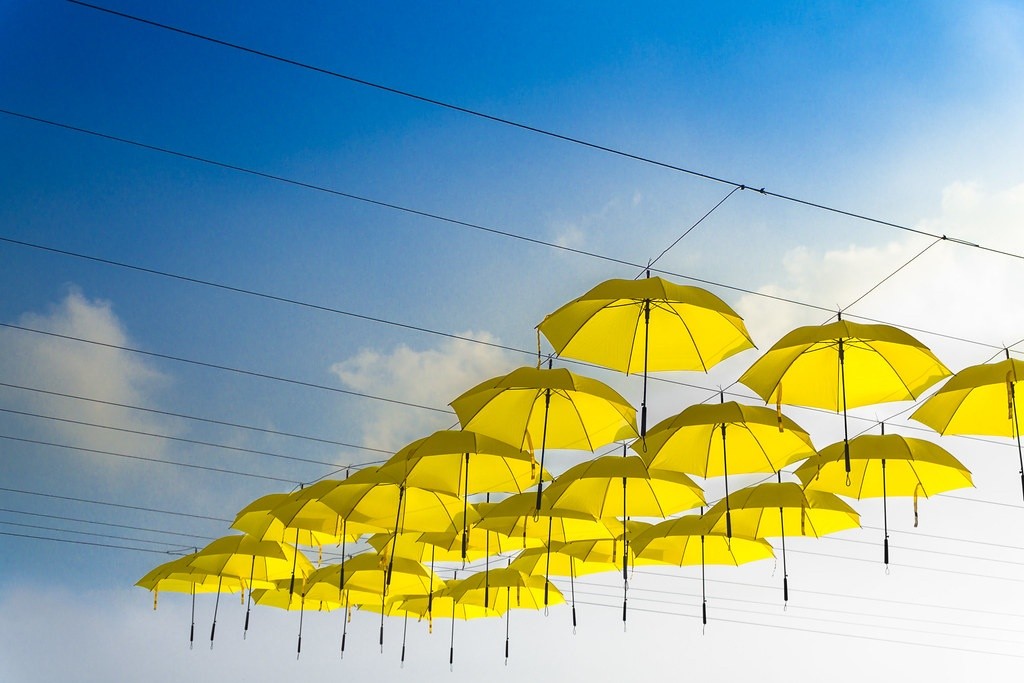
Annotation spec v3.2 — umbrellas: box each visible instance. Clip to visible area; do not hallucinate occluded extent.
[701,471,862,609]
[323,547,631,673]
[541,441,707,635]
[631,391,821,550]
[474,480,631,617]
[629,513,777,634]
[229,435,553,601]
[249,552,566,673]
[792,422,976,575]
[447,357,641,522]
[907,347,1024,499]
[618,514,655,588]
[533,270,758,453]
[133,533,316,651]
[375,429,555,560]
[737,312,958,486]
